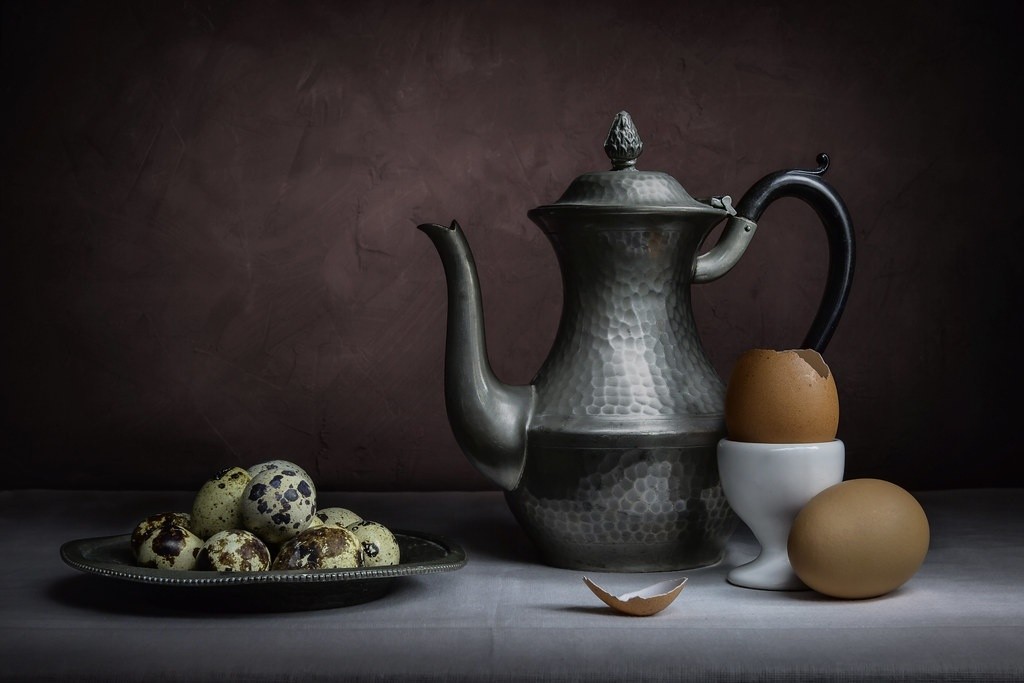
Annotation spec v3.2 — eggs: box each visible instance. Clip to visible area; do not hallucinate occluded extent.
[724,348,930,603]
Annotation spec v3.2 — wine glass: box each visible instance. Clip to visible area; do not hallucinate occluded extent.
[715,432,845,590]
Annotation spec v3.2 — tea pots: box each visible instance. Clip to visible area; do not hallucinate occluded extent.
[417,104,863,565]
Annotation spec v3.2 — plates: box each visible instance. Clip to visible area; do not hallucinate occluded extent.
[57,526,466,587]
